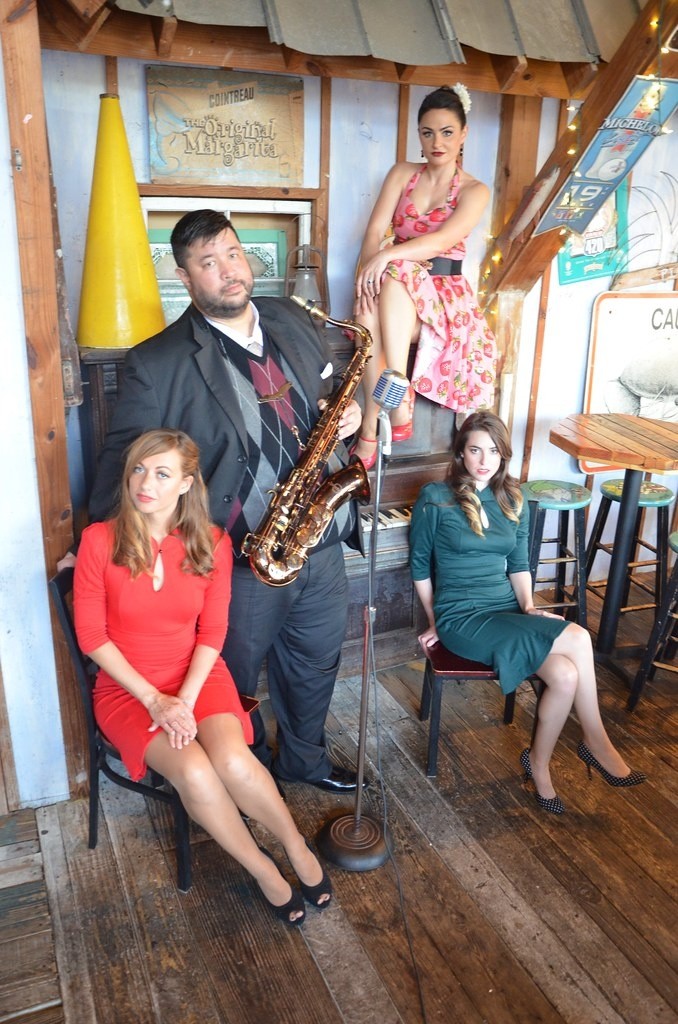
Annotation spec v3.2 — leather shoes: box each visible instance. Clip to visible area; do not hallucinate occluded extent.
[315,765,370,795]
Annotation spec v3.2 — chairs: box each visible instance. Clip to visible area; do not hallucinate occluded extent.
[50,567,261,894]
[418,551,547,777]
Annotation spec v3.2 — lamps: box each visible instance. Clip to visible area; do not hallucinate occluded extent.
[284,212,332,336]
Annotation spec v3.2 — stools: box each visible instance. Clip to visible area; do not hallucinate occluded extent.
[520,480,592,629]
[562,479,676,624]
[625,531,678,712]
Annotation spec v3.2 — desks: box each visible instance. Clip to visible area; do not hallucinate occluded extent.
[549,412,678,689]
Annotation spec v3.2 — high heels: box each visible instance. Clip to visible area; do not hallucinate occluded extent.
[521,748,566,815]
[578,741,647,786]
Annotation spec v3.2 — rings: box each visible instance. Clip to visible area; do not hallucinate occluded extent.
[181,712,186,717]
[367,280,373,283]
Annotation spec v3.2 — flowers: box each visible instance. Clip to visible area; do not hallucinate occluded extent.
[452,83,472,115]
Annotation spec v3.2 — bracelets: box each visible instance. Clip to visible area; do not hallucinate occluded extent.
[181,696,195,709]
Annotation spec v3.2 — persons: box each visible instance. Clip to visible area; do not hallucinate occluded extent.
[55,209,371,806]
[73,427,333,926]
[345,84,498,473]
[408,412,646,818]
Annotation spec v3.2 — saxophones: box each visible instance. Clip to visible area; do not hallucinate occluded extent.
[238,292,377,592]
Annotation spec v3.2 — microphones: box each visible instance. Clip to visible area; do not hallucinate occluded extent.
[372,369,410,456]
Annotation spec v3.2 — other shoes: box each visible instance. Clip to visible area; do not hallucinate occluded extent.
[392,386,417,441]
[349,436,378,470]
[254,847,308,926]
[282,839,332,910]
